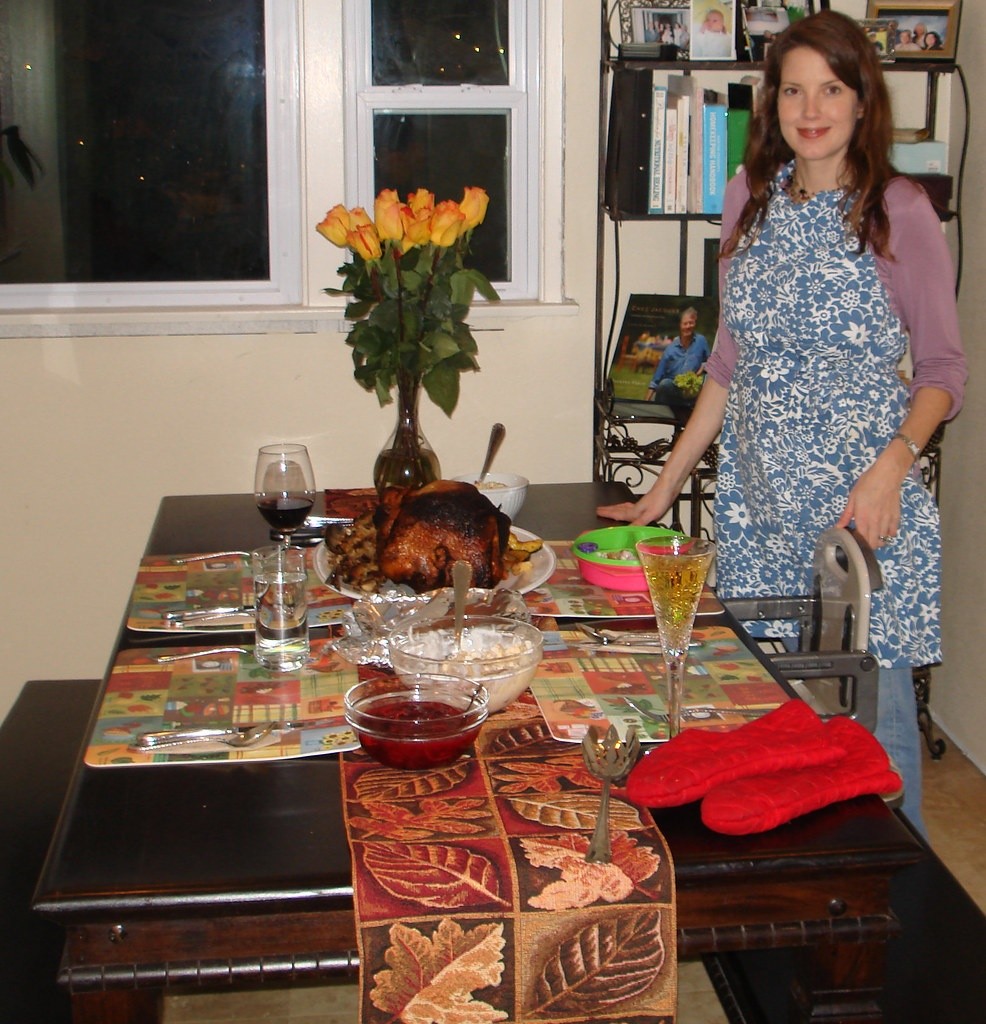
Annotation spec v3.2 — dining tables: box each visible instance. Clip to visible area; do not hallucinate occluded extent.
[29,481,930,1024]
[629,340,667,373]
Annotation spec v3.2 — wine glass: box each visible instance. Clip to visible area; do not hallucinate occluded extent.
[252,440,318,557]
[635,535,717,756]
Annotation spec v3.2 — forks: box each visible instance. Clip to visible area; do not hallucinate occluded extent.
[622,694,778,725]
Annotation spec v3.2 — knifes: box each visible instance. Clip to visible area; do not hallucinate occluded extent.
[138,717,316,747]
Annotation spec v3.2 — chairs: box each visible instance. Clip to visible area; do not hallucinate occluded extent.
[615,335,639,373]
[716,528,906,814]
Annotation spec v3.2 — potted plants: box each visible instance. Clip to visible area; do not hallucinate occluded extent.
[673,370,704,400]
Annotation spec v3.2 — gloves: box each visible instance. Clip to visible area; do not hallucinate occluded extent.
[702,715,902,834]
[628,698,847,806]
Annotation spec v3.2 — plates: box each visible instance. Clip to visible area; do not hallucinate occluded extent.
[571,526,699,592]
[311,522,557,602]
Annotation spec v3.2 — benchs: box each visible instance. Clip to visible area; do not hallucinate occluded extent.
[707,808,986,1023]
[0,679,99,1022]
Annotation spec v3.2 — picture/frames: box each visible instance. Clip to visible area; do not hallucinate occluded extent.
[631,0,963,63]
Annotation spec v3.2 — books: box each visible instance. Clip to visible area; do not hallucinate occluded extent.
[883,125,954,215]
[648,72,764,214]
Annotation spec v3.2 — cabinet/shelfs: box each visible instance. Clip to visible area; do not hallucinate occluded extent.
[591,0,974,761]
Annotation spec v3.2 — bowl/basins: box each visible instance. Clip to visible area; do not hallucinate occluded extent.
[451,470,529,522]
[344,672,490,770]
[388,614,544,715]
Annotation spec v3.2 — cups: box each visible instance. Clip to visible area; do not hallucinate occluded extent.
[252,544,311,673]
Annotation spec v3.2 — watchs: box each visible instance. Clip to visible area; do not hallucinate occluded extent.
[892,433,922,465]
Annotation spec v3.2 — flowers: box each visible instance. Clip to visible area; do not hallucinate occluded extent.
[315,184,503,419]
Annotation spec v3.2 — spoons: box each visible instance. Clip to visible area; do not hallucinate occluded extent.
[573,621,704,646]
[173,541,283,564]
[439,559,474,677]
[135,721,278,751]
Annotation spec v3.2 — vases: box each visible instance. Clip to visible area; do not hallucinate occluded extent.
[373,369,443,491]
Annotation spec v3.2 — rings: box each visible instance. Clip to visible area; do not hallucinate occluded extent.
[879,535,895,545]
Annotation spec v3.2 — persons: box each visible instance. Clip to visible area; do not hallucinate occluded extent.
[690,9,732,58]
[867,16,943,58]
[643,20,690,54]
[594,8,970,846]
[753,30,778,63]
[648,305,711,403]
